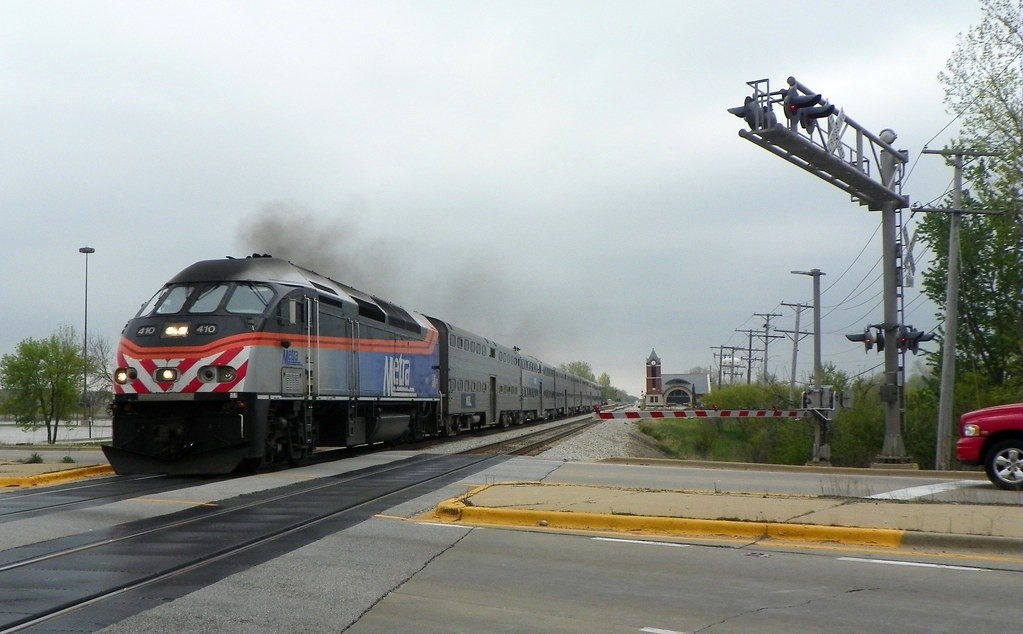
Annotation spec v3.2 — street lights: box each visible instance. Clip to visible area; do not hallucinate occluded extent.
[78,246,95,400]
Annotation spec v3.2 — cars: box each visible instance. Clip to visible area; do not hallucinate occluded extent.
[955,402,1023,491]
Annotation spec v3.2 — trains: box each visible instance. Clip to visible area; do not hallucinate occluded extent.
[100,253,611,479]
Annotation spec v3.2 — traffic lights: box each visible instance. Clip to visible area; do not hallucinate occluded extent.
[845,332,873,354]
[899,325,924,352]
[726,97,758,132]
[912,327,935,355]
[800,103,836,135]
[788,87,823,116]
